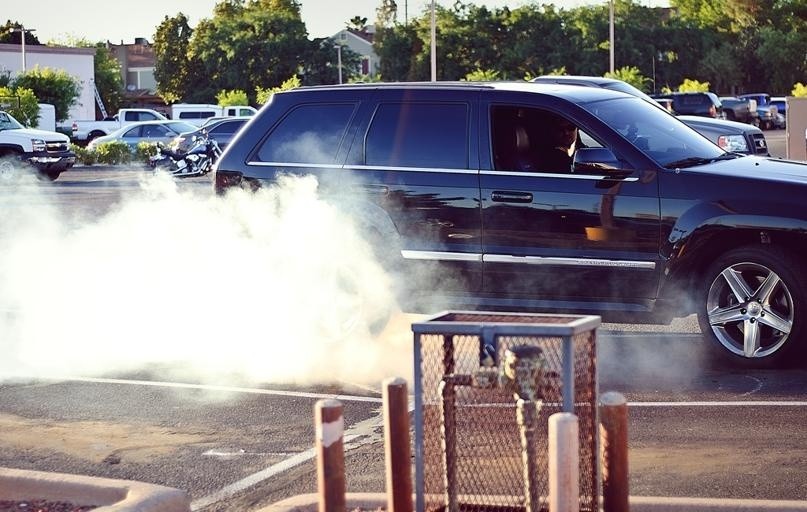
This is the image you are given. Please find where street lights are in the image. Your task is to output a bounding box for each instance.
[333,45,343,84]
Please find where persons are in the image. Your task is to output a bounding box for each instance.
[533,112,614,174]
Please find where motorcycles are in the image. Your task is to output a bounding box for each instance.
[144,127,223,181]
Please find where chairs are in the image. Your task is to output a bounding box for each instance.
[507,124,539,170]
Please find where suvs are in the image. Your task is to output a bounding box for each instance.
[0,96,76,183]
[208,80,807,372]
[531,73,771,158]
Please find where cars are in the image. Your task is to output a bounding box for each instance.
[651,92,786,129]
[69,102,258,162]
